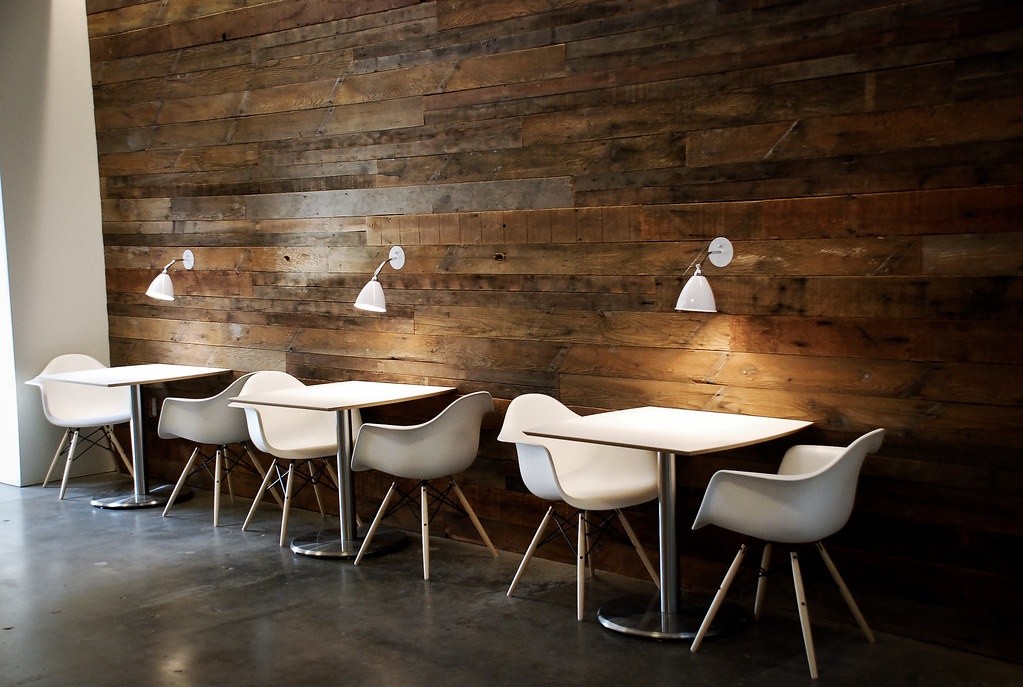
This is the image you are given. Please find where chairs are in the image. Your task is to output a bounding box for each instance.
[686,428,886,679]
[228,370,363,548]
[496,393,660,622]
[158,372,285,527]
[349,390,500,581]
[24,353,135,500]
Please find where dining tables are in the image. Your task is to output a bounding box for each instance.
[37,362,234,511]
[227,379,457,557]
[523,405,816,637]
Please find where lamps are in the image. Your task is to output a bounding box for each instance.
[673,236,734,313]
[144,249,195,301]
[353,245,406,313]
